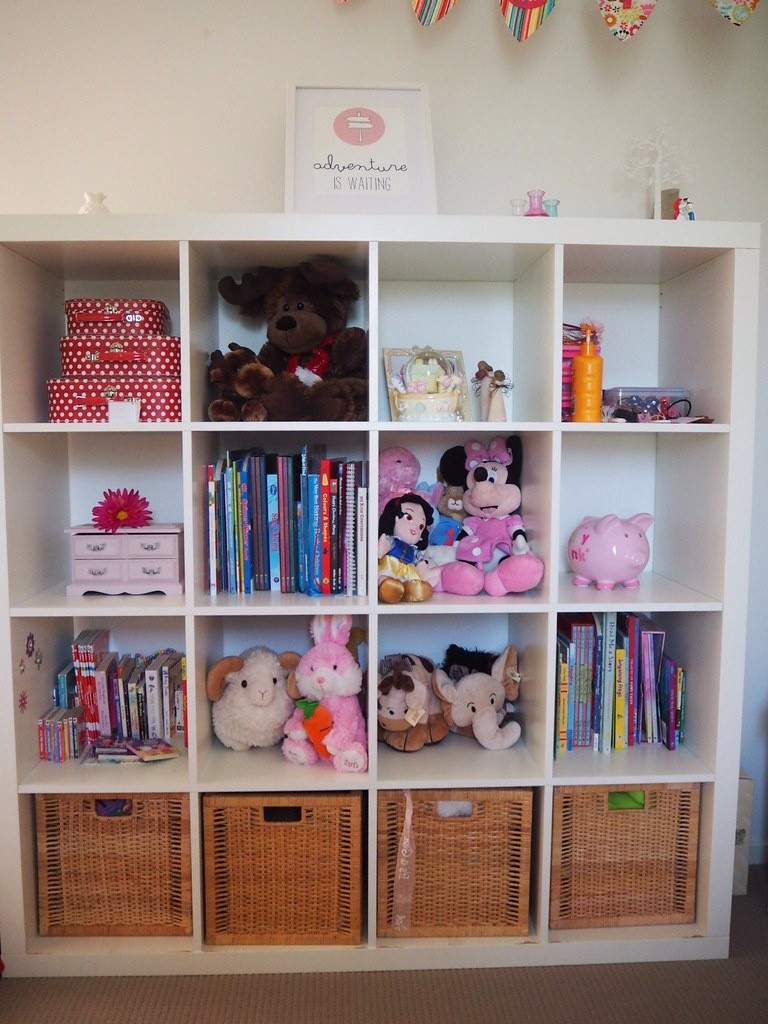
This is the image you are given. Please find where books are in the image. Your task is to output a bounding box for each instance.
[36,629,187,766]
[556,612,686,758]
[204,442,369,601]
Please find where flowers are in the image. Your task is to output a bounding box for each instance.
[90,488,154,531]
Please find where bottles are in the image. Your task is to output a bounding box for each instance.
[525,189,549,216]
[543,200,561,216]
[76,188,109,214]
[510,197,527,215]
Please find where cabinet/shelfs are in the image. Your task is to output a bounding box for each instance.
[0,217,761,983]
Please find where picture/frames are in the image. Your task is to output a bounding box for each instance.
[382,346,472,422]
[285,81,436,215]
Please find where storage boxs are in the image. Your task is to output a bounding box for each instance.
[66,524,183,596]
[731,770,754,896]
[604,386,690,417]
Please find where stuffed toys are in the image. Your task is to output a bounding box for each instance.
[204,614,367,774]
[376,643,522,753]
[206,254,370,422]
[377,435,543,604]
